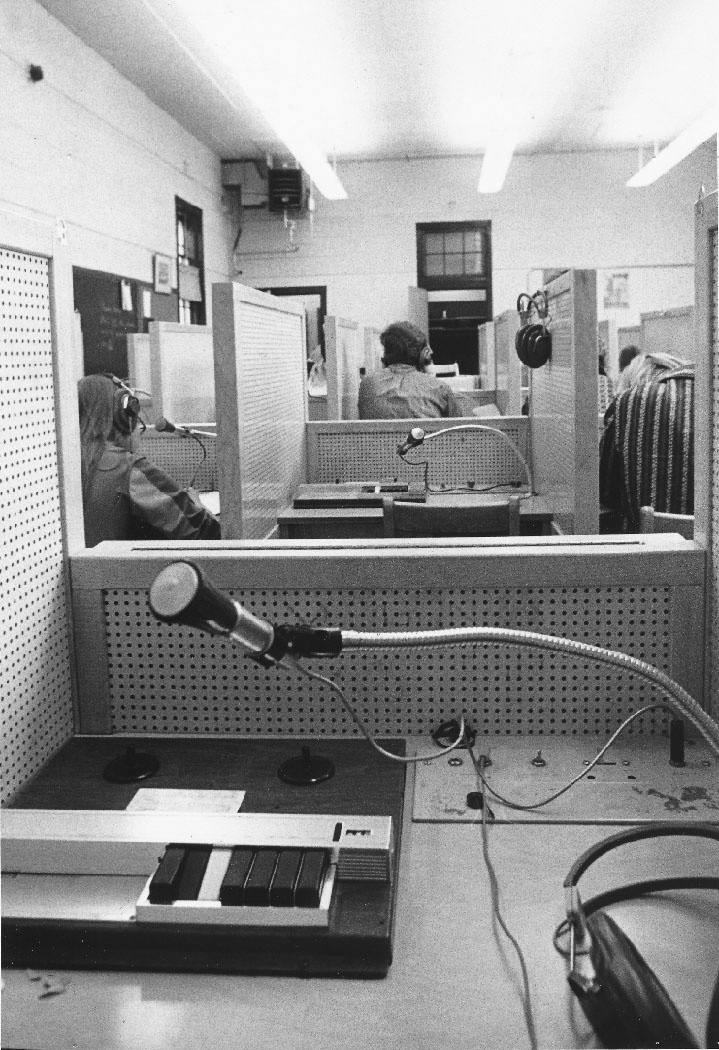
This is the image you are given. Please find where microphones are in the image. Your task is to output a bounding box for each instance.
[154,416,176,434]
[397,427,425,455]
[148,560,286,662]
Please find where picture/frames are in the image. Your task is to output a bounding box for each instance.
[153,252,172,295]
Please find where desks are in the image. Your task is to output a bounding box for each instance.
[278,484,554,538]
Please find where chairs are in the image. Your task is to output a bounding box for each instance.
[639,506,694,541]
[382,496,521,536]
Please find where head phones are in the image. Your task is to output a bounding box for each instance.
[552,820,719,1048]
[514,290,552,368]
[416,341,433,372]
[102,372,140,436]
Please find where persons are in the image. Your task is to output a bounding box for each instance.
[358,322,463,421]
[77,375,220,550]
[614,346,643,397]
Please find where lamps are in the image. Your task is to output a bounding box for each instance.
[266,103,348,201]
[477,113,517,193]
[625,102,719,189]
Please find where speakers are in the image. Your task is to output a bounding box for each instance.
[267,169,303,211]
